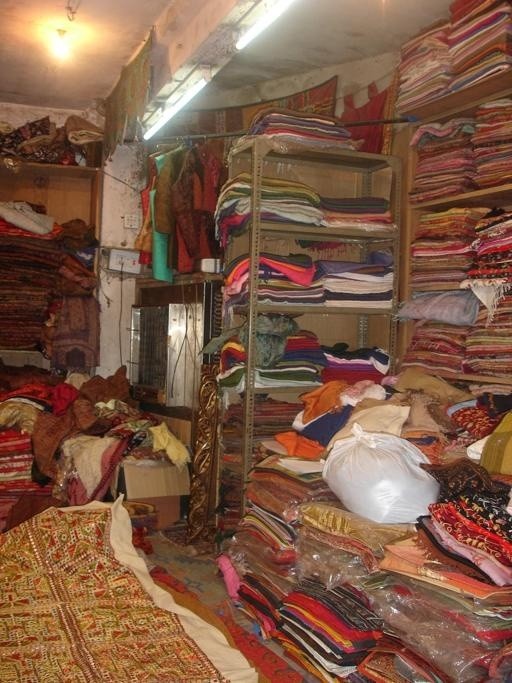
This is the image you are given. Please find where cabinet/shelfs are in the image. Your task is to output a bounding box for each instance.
[210,84,512,533]
[1,158,102,379]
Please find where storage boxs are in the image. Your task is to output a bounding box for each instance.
[111,458,192,532]
